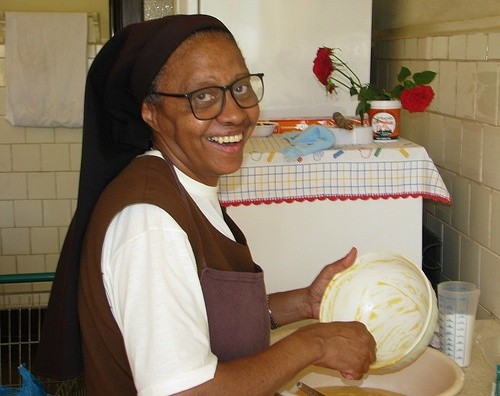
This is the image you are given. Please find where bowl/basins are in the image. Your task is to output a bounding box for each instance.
[275,346,465,396]
[250,120,279,136]
[319,250,437,376]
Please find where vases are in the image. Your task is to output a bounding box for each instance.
[364,99,402,141]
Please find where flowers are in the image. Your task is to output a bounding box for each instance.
[312,45,436,123]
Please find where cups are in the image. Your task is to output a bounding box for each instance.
[438,279,481,368]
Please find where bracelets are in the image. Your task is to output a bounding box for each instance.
[266,293,279,330]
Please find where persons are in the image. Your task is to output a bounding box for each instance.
[31,14,376,396]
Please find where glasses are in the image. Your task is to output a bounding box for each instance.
[153,73,264,121]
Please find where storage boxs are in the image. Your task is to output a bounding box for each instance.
[330,127,374,145]
